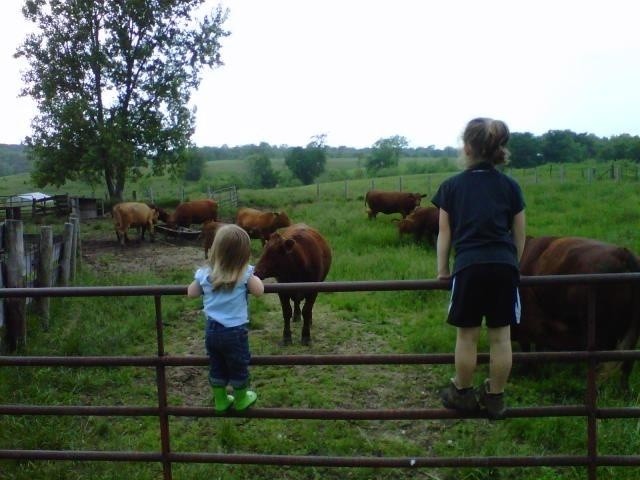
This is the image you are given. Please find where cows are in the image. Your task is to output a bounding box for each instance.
[140,203,171,241]
[202,220,251,260]
[363,190,427,222]
[510,235,640,396]
[253,222,332,347]
[237,208,291,248]
[111,202,159,249]
[168,199,219,230]
[390,205,440,248]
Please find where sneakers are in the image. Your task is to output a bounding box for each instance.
[479,378,508,421]
[438,378,480,416]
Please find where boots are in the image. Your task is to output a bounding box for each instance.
[212,384,235,414]
[232,385,258,412]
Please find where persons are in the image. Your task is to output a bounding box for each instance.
[429,118,526,416]
[184,223,265,413]
